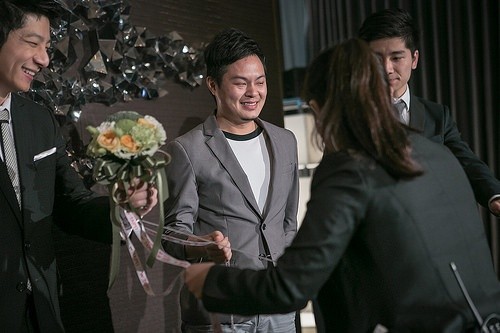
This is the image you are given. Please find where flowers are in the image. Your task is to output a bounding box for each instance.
[86,109,173,292]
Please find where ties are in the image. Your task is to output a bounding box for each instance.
[394,102,406,124]
[0,111,21,209]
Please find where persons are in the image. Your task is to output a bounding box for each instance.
[0,0,160,333]
[161,27,299,333]
[182,36,500,333]
[358,9,500,220]
